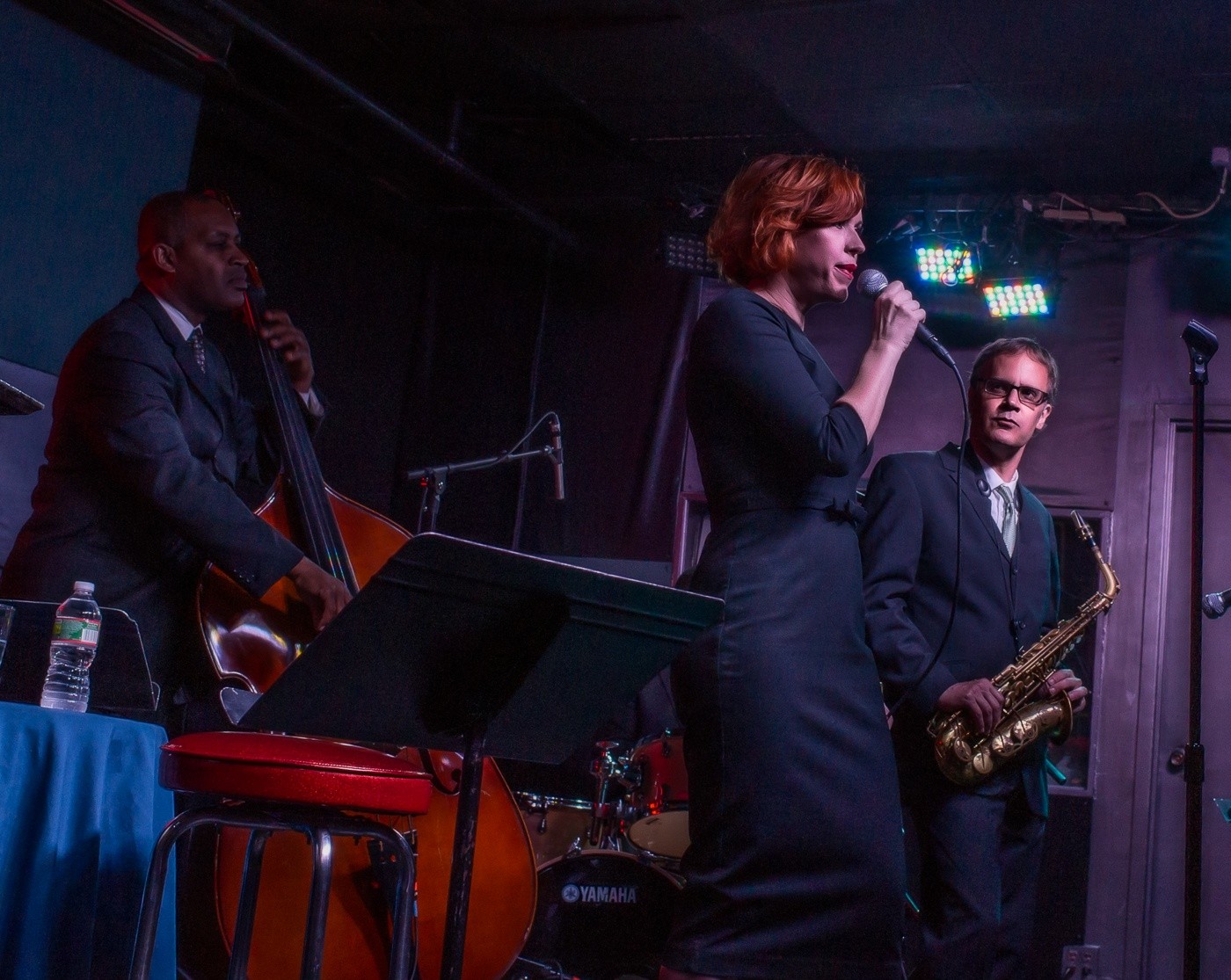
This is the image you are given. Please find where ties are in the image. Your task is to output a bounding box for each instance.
[998,485,1017,557]
[191,331,209,374]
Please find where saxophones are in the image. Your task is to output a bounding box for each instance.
[937,510,1122,785]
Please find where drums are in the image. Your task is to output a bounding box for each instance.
[622,734,691,863]
[500,848,688,980]
[508,788,615,870]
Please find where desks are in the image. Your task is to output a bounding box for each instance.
[0,700,177,980]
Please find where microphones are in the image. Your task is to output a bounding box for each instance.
[550,414,564,499]
[857,269,956,368]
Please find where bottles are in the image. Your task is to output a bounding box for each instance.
[40,580,103,712]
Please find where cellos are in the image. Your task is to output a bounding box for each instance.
[183,189,539,980]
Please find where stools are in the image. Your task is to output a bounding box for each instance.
[129,728,427,979]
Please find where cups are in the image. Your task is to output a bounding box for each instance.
[0,605,15,664]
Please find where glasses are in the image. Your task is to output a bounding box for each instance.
[979,378,1050,406]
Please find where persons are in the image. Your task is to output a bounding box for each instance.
[863,338,1088,980]
[658,156,927,980]
[0,189,354,726]
